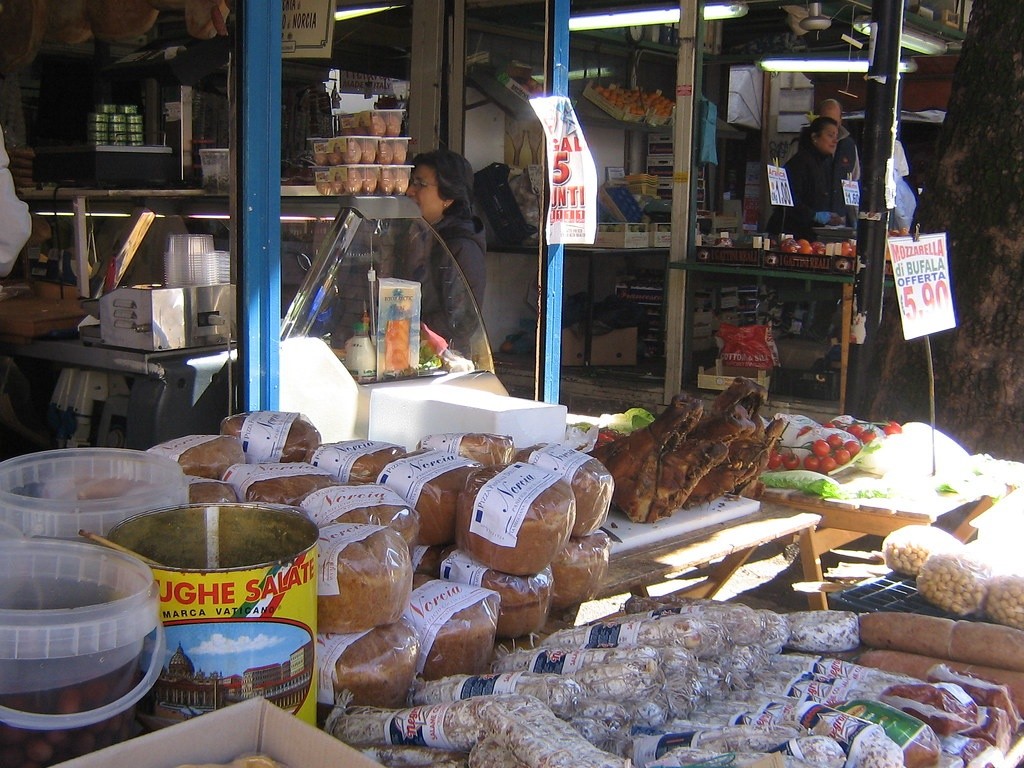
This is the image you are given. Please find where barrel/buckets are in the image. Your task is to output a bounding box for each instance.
[107,502,320,728]
[0,538,166,768]
[0,448,190,543]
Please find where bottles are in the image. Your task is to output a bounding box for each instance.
[346,321,376,385]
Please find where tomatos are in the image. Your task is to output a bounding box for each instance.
[765,419,903,474]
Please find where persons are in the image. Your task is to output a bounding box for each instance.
[765,100,861,343]
[0,123,53,277]
[396,150,486,360]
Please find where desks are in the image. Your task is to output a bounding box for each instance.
[763,469,1011,611]
[596,503,828,611]
[668,258,855,424]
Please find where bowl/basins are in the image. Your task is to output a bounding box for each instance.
[165,233,232,287]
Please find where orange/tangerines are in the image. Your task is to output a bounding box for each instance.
[595,86,674,116]
[780,237,826,255]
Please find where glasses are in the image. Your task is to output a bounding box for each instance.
[411,178,438,190]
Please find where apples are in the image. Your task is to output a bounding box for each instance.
[600,215,672,232]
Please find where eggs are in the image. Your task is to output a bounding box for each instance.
[313,113,409,196]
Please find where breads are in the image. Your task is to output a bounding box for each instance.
[73,411,616,711]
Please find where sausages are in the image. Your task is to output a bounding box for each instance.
[328,602,1024,768]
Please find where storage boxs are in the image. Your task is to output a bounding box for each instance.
[743,162,761,232]
[646,133,705,211]
[561,321,638,368]
[695,243,762,267]
[369,384,568,447]
[649,223,703,247]
[763,248,856,275]
[49,696,385,767]
[691,282,821,392]
[496,67,542,103]
[583,79,672,124]
[305,108,416,197]
[593,222,651,249]
[933,9,961,29]
[630,183,657,198]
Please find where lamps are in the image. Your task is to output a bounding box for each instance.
[568,0,749,32]
[852,15,948,56]
[756,54,918,73]
